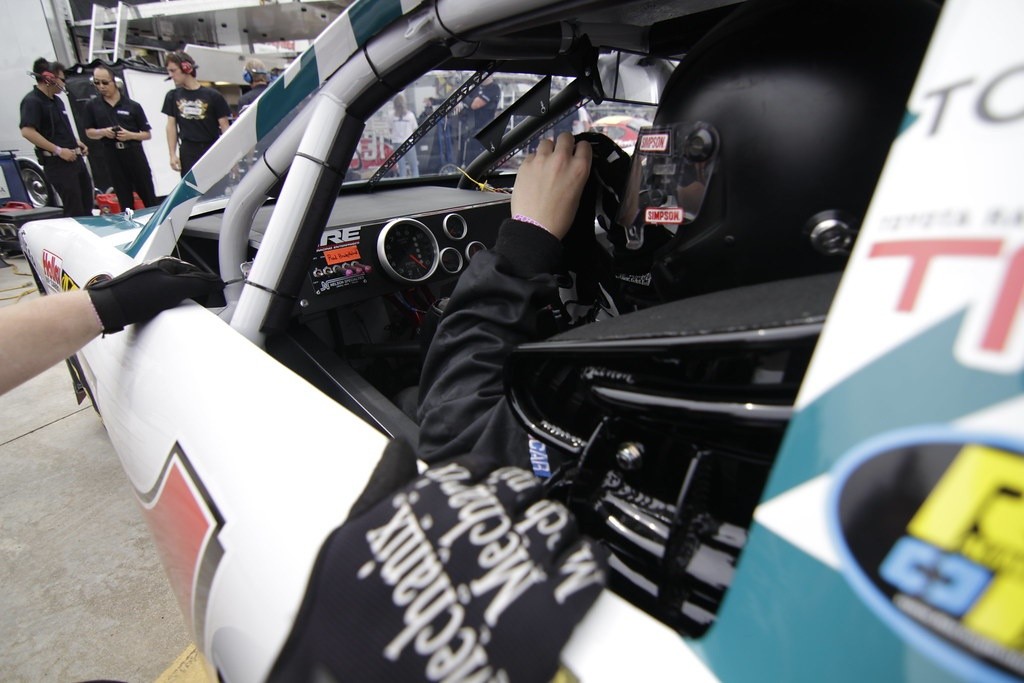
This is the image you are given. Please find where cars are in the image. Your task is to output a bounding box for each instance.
[18,0,1024,683]
[588,116,653,157]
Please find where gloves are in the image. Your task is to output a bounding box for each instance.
[87,257,227,334]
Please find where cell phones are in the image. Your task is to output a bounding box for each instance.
[112,126,121,131]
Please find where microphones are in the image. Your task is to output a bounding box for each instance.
[164,76,172,81]
[52,80,69,95]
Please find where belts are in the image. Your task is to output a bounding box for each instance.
[43,148,83,158]
[105,140,141,148]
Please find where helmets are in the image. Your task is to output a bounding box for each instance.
[651,4,919,290]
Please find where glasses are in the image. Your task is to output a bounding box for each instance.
[56,75,65,83]
[92,76,114,85]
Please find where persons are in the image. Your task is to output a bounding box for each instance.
[0,255,226,396]
[19,58,94,217]
[236,58,271,116]
[415,0,941,487]
[160,51,231,181]
[84,64,158,213]
[554,101,590,142]
[461,72,501,129]
[391,93,419,177]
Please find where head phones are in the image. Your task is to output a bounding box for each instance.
[42,62,56,85]
[173,52,194,73]
[89,66,124,89]
[242,68,273,83]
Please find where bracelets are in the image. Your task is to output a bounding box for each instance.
[55,146,61,155]
[96,129,102,139]
